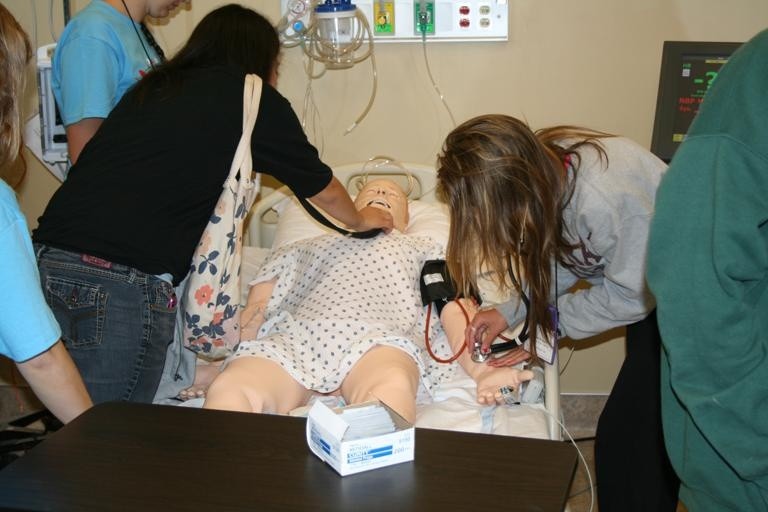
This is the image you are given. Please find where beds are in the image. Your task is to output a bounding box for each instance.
[155,163,571,512]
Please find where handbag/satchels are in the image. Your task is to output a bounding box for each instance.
[180,176,255,362]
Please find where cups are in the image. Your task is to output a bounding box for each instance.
[313,1,356,70]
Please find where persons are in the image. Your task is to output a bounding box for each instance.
[174,176,536,427]
[29,4,394,404]
[47,0,194,170]
[639,31,767,512]
[434,109,679,510]
[0,2,95,428]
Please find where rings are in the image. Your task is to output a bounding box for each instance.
[470,327,477,332]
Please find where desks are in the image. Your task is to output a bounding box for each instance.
[0,402,580,512]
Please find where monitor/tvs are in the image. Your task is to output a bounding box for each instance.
[650,41,746,164]
